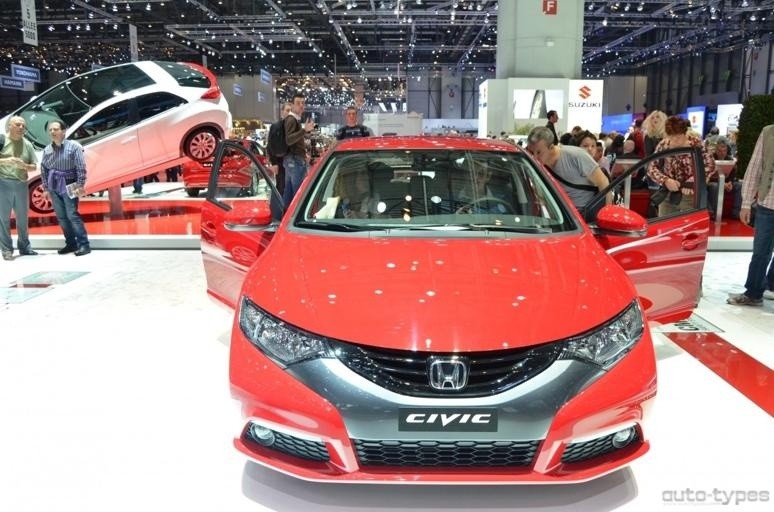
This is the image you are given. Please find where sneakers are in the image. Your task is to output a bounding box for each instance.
[763,289,774,300]
[18,249,39,256]
[727,295,764,307]
[2,250,15,260]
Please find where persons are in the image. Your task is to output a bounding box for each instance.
[86,165,181,197]
[455,158,508,214]
[527,110,774,308]
[270,102,293,223]
[313,192,381,219]
[0,116,38,260]
[40,119,91,256]
[282,93,316,214]
[336,106,369,140]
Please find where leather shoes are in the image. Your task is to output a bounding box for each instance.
[74,243,91,256]
[58,243,78,254]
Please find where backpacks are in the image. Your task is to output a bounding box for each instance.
[268,114,298,158]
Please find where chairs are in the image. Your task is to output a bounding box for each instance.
[410,177,432,211]
[345,162,409,221]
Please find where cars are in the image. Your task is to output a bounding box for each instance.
[181,135,269,196]
[197,132,717,490]
[0,55,236,216]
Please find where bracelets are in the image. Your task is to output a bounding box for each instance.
[367,211,371,219]
